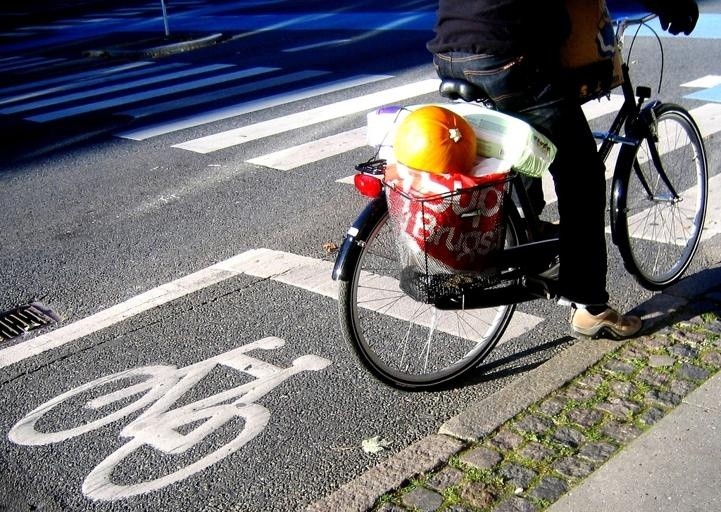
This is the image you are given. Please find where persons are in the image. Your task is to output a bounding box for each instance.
[426,0,698,339]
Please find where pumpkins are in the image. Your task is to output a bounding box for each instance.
[393,104,478,174]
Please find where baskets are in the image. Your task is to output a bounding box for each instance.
[381,171,515,302]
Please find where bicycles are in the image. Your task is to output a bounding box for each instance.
[331,10,708,391]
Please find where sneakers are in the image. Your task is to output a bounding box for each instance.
[568,307,642,342]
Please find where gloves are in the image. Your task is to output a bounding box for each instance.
[649,2,699,35]
